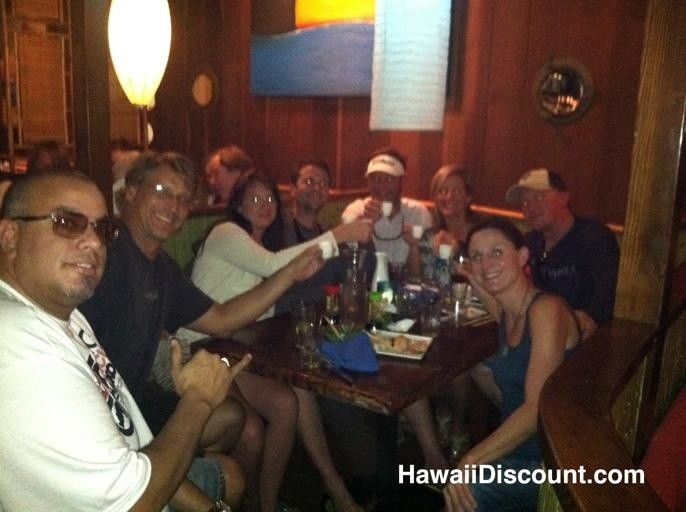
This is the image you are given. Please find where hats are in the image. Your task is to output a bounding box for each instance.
[504,167,566,204]
[363,153,404,178]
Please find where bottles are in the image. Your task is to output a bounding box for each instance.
[292,249,366,367]
[369,250,394,317]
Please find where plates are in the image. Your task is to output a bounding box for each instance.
[364,329,434,362]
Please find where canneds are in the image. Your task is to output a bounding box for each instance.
[321,284,341,327]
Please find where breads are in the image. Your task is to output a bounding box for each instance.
[407,339,428,355]
[391,334,408,353]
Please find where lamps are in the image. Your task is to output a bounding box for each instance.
[369,0,452,130]
[108,0,172,151]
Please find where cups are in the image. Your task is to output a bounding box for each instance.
[381,202,393,215]
[318,240,333,260]
[403,274,476,338]
[411,225,424,240]
[438,244,453,261]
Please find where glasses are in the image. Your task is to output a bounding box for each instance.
[242,194,275,206]
[142,181,195,211]
[9,210,120,246]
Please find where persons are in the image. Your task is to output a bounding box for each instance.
[1,136,622,511]
